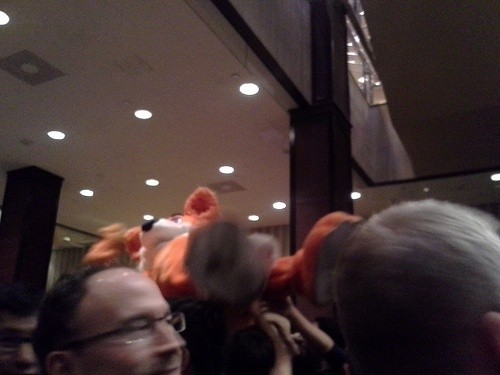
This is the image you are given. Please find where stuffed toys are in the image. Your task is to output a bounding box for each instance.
[80,186,365,309]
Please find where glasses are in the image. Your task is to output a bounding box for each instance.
[72,312,186,347]
[1,333,34,351]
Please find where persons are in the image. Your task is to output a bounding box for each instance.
[0,199,500,375]
